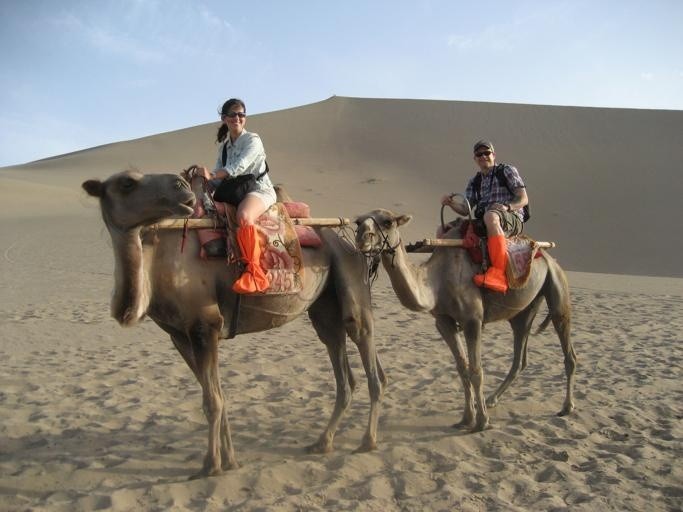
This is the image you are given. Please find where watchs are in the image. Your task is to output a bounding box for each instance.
[210,172,217,180]
[503,203,511,212]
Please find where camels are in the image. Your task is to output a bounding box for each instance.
[81,170,387,482]
[349,208,577,432]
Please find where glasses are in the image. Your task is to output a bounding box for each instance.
[225,111,246,118]
[474,151,493,157]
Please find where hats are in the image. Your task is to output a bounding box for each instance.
[474,139,494,151]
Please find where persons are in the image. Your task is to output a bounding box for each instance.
[196,98,276,293]
[436,138,530,293]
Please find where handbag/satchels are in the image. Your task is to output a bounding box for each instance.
[212,173,261,204]
